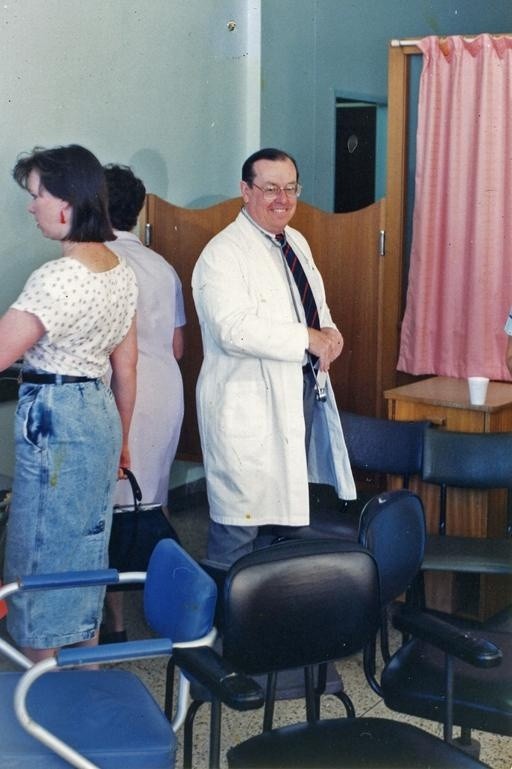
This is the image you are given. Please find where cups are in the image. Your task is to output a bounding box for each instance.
[468,375,491,406]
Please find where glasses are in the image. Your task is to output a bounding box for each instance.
[250,181,302,200]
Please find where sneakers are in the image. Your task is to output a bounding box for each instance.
[97,624,130,646]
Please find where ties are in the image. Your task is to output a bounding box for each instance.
[276,233,324,332]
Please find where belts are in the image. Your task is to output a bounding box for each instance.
[13,371,96,383]
[299,357,320,374]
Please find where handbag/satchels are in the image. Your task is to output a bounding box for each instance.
[105,468,182,593]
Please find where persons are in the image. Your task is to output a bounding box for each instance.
[102,163,186,643]
[190,148,359,565]
[1,144,138,674]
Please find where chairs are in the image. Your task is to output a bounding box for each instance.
[165,536,355,769]
[356,489,512,759]
[411,422,511,616]
[280,410,433,647]
[0,538,217,769]
[171,540,505,768]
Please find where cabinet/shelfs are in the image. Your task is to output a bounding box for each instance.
[383,376,512,624]
